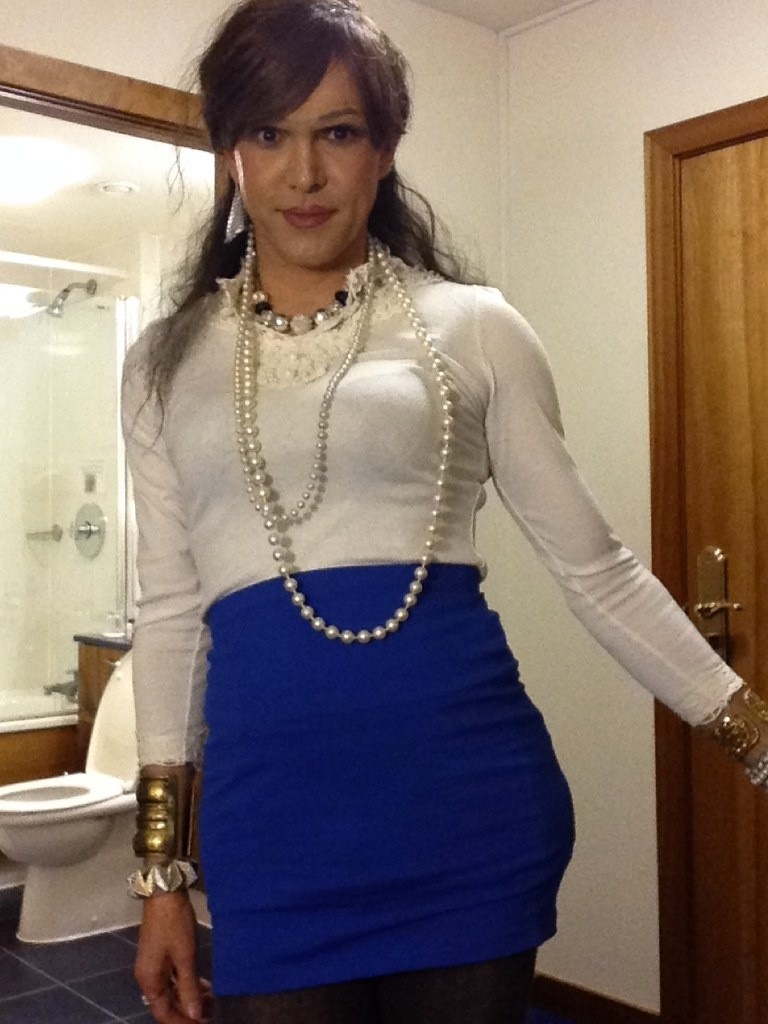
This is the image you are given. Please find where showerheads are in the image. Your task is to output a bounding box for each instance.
[46,288,71,320]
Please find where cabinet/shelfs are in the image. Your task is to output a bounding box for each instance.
[73,642,202,893]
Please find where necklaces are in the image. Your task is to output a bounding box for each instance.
[232,218,453,643]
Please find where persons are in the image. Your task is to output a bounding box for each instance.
[120,0,768,1024]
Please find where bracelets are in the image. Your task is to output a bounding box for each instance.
[711,688,768,785]
[125,777,199,899]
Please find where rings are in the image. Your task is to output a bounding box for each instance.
[142,987,166,1005]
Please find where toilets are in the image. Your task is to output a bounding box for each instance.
[0,646,145,945]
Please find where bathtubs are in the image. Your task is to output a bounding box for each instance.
[0,687,80,786]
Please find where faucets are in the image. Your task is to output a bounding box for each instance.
[43,668,79,704]
